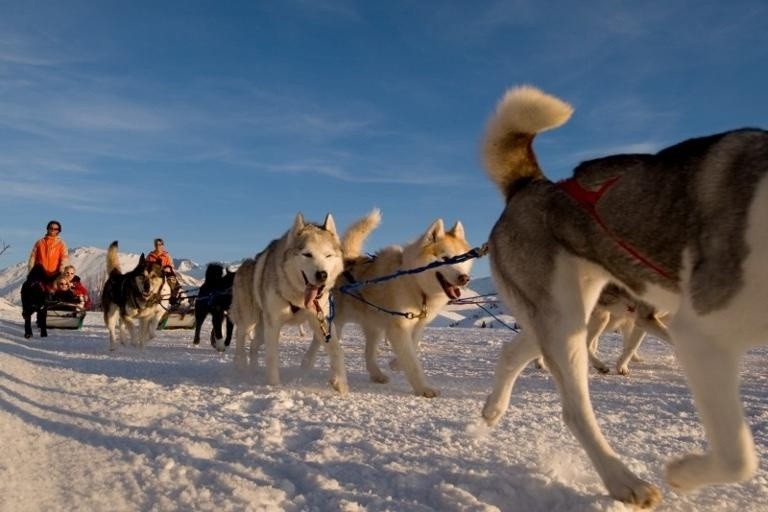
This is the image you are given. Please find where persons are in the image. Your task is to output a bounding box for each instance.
[145,238,174,272]
[28,220,92,310]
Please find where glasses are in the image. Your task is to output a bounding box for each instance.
[50,228,59,230]
[65,272,74,276]
[58,283,69,285]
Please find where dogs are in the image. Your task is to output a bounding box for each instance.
[193,263,250,348]
[469,89,768,510]
[299,206,475,391]
[99,239,172,352]
[230,213,353,398]
